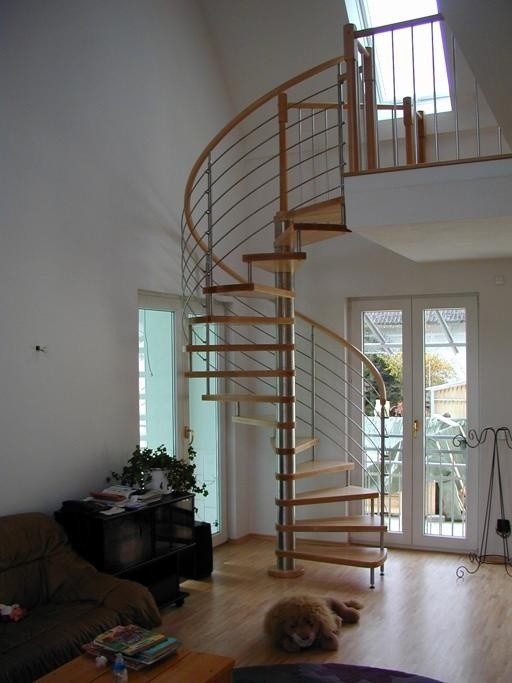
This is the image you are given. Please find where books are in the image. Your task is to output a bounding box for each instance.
[80,484,164,516]
[81,623,184,672]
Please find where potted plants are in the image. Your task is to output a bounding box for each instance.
[106,444,209,497]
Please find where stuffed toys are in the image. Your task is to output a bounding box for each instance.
[262,592,365,652]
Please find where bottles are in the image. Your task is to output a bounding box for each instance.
[112,653,128,683]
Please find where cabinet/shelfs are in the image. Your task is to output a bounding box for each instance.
[63,490,195,609]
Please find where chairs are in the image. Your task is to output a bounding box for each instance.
[1,512,148,682]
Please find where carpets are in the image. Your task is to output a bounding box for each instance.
[233,663,445,683]
